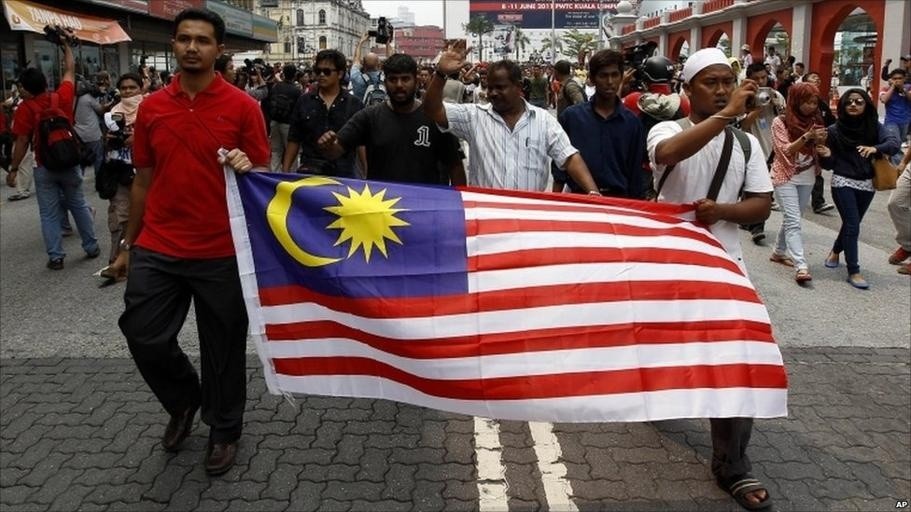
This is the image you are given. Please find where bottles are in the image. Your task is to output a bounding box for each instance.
[899,53,911,61]
[740,43,751,52]
[682,47,733,84]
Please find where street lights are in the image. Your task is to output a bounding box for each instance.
[708,127,732,205]
[873,158,897,190]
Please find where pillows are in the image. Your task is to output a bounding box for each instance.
[642,56,675,83]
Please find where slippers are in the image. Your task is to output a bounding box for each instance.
[25,91,86,173]
[362,71,390,107]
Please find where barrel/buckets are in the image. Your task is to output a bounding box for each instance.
[795,268,813,282]
[716,470,773,511]
[769,253,795,267]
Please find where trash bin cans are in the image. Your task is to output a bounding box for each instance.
[8,164,20,172]
[119,239,130,250]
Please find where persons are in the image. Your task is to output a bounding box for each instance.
[267,65,305,171]
[803,72,834,212]
[887,128,911,281]
[738,44,751,71]
[620,53,692,132]
[509,56,591,102]
[216,54,237,84]
[794,62,803,90]
[103,9,271,475]
[295,60,317,90]
[522,65,548,108]
[93,74,151,278]
[784,55,805,86]
[320,55,465,186]
[67,74,105,192]
[136,65,175,93]
[470,70,489,103]
[234,75,266,100]
[419,67,427,85]
[553,58,586,193]
[830,58,879,88]
[8,83,40,200]
[815,89,896,287]
[349,31,392,179]
[766,45,789,79]
[426,38,603,197]
[647,50,779,509]
[7,41,99,268]
[281,49,368,177]
[247,67,263,103]
[460,58,485,89]
[881,55,909,79]
[767,82,827,284]
[883,68,908,160]
[549,50,652,200]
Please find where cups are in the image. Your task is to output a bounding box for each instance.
[313,67,339,77]
[845,97,866,106]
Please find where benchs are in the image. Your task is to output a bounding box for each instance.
[216,147,790,423]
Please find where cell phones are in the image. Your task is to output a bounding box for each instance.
[751,88,772,107]
[242,59,273,80]
[77,80,108,98]
[369,17,393,44]
[624,41,657,90]
[41,25,82,47]
[108,112,130,150]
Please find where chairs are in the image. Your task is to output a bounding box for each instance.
[61,227,74,238]
[738,224,750,230]
[896,260,911,275]
[768,201,780,211]
[750,232,766,242]
[7,188,31,201]
[90,207,98,222]
[814,202,834,213]
[888,249,910,265]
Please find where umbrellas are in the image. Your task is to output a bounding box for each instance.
[159,394,196,454]
[825,248,839,267]
[100,264,124,280]
[86,244,101,259]
[847,273,869,289]
[200,428,239,478]
[46,257,64,271]
